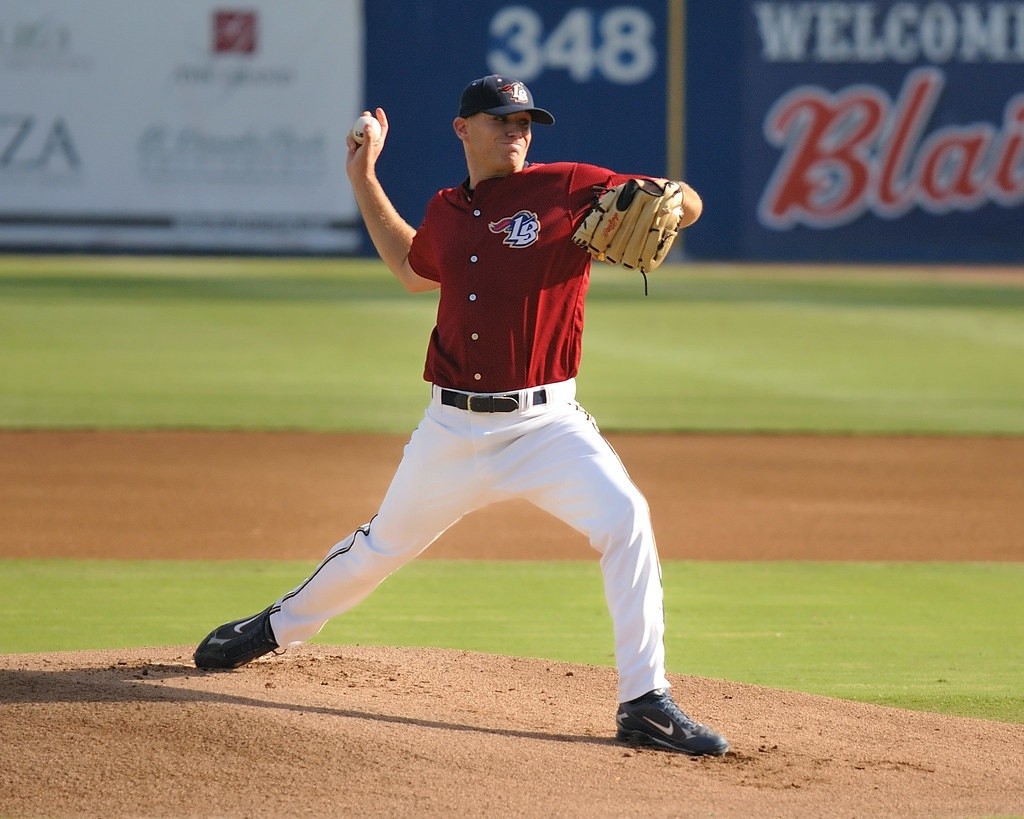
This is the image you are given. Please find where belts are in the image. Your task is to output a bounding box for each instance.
[442,389,547,413]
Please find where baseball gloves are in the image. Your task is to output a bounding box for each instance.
[569,176,686,296]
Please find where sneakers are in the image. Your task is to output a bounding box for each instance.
[194,602,285,668]
[615,688,730,754]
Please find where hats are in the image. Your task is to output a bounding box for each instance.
[459,74,555,124]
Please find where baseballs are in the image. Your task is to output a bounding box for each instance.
[352,115,382,144]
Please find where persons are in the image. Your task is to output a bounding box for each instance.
[194,74,728,756]
